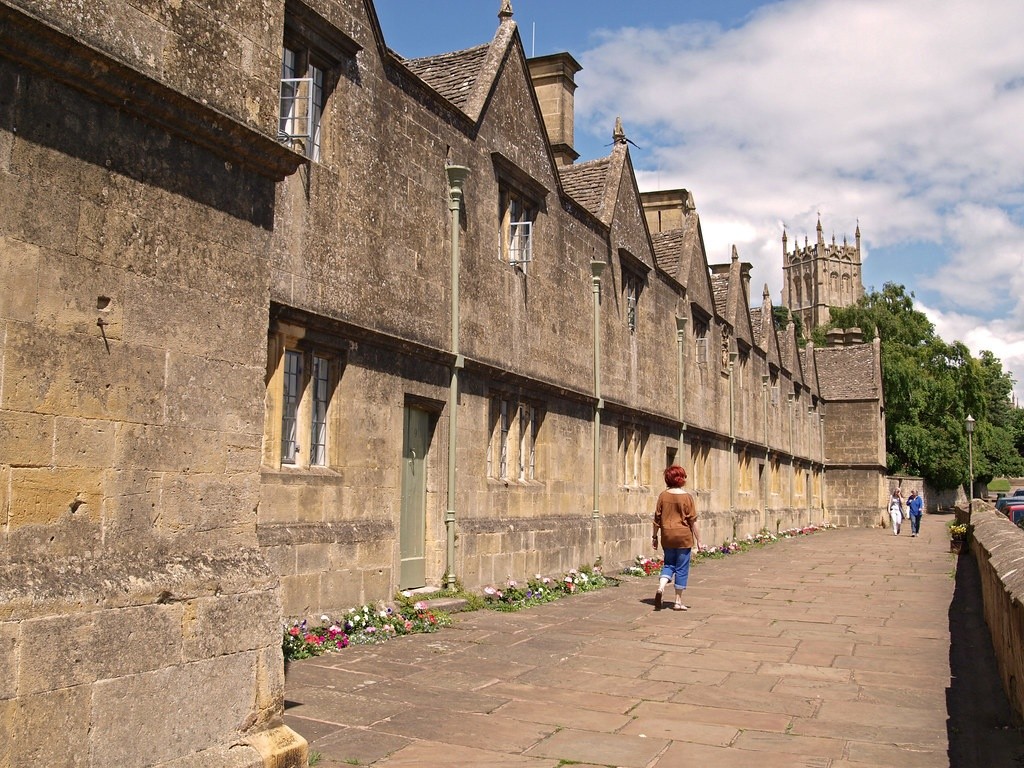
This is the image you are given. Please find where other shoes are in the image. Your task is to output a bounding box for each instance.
[894,533,897,536]
[897,530,900,534]
[654,587,663,611]
[916,534,919,537]
[673,602,688,611]
[912,533,915,537]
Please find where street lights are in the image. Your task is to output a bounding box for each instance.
[964,414,975,500]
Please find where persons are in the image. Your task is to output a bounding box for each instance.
[888,487,904,536]
[652,465,701,611]
[906,489,923,537]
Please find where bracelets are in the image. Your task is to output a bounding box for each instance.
[652,536,657,539]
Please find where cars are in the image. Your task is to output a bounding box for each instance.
[996,487,1024,526]
